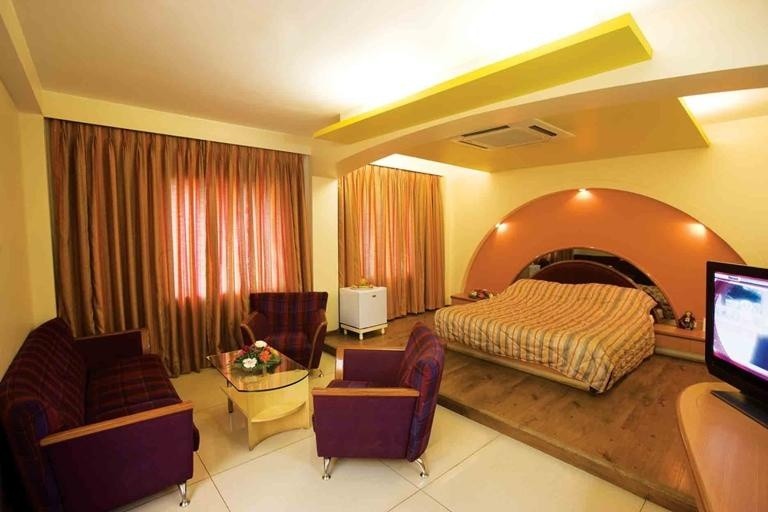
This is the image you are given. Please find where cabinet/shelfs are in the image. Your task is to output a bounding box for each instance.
[339,285,388,342]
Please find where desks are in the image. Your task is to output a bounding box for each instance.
[675,379,767,512]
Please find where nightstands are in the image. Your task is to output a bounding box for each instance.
[654,321,707,362]
[449,292,482,308]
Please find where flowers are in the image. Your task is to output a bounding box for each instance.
[229,340,281,376]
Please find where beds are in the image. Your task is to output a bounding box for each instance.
[434,256,657,397]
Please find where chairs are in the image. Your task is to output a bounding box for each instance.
[312,321,443,480]
[236,289,328,379]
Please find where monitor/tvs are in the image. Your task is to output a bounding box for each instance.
[704,260,768,430]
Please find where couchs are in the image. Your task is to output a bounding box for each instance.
[1,316,199,511]
